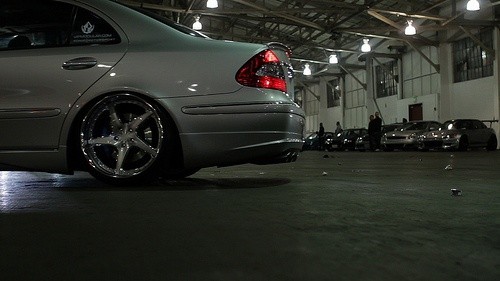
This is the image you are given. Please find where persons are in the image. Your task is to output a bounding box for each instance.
[317,123,324,151]
[367,112,382,151]
[336,121,341,132]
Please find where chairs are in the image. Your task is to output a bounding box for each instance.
[8,35,31,50]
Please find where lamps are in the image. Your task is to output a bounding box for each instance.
[328,52,338,64]
[361,37,371,52]
[192,13,202,30]
[303,62,312,75]
[405,18,416,35]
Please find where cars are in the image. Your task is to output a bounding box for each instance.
[417,119,498,151]
[304,122,416,152]
[381,121,443,152]
[0,0,306,185]
